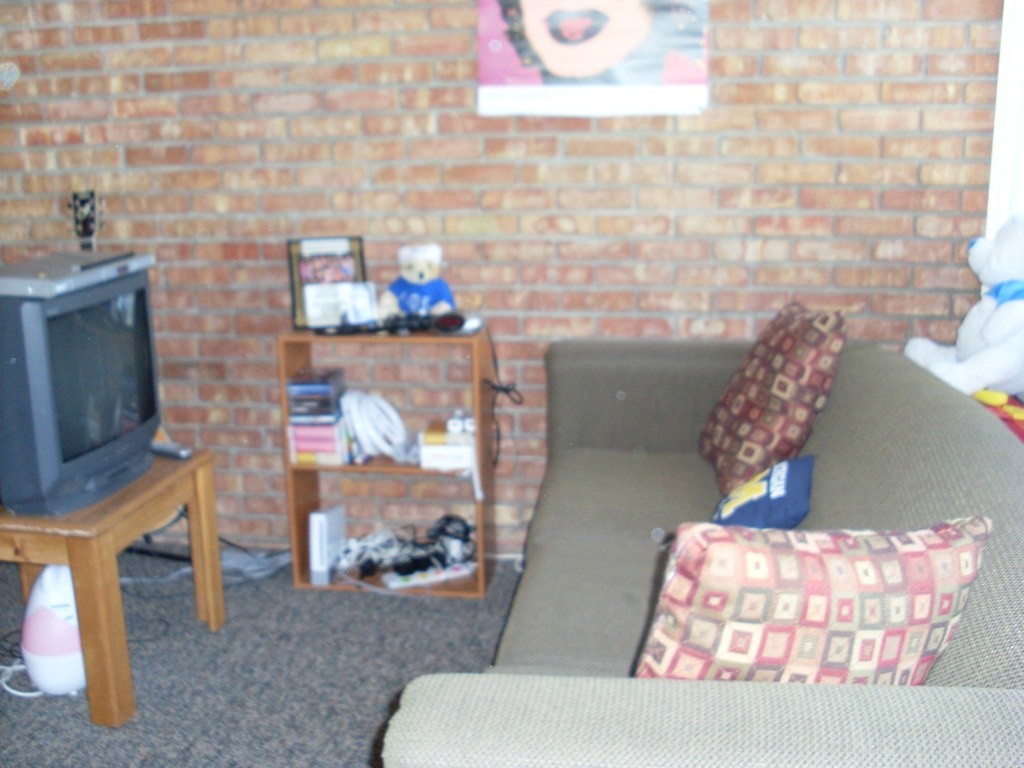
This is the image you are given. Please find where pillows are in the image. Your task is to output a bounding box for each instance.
[699,300,848,496]
[633,514,992,688]
[708,456,816,527]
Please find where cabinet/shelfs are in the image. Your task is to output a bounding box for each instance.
[279,328,491,596]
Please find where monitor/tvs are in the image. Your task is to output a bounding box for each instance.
[0,270,162,518]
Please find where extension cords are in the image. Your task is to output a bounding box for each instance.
[382,562,476,590]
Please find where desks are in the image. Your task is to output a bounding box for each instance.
[0,449,225,726]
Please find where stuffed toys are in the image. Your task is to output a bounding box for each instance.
[378,244,465,333]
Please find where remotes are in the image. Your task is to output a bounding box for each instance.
[151,443,194,460]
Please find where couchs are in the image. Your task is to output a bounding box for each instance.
[379,339,1024,768]
